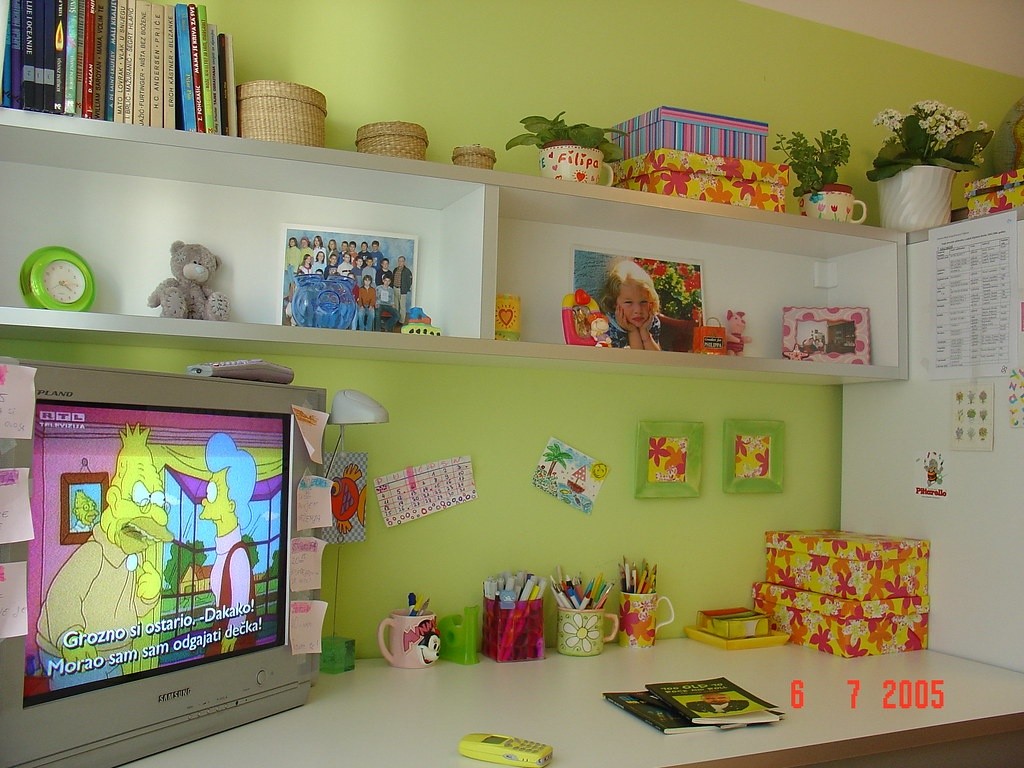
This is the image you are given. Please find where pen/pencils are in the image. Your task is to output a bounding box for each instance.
[548,565,582,609]
[419,599,430,616]
[581,573,614,610]
[617,555,657,594]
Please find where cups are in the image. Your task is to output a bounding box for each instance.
[556,606,619,656]
[616,592,675,647]
[481,598,545,662]
[378,608,442,668]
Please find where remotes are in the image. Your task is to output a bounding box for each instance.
[188,359,295,385]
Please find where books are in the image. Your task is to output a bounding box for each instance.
[0,0,240,137]
[600,675,786,737]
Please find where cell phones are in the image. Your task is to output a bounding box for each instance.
[457,733,553,768]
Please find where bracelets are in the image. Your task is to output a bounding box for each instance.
[643,334,652,342]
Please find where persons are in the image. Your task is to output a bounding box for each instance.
[802,337,827,354]
[685,692,749,713]
[597,261,663,351]
[284,235,412,332]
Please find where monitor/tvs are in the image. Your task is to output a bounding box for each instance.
[0,356,319,768]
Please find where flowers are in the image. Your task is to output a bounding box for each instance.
[865,100,996,182]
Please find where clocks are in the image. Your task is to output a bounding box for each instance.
[19,245,95,311]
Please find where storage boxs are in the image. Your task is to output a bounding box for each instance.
[610,106,769,162]
[766,530,929,600]
[610,148,789,215]
[752,581,929,658]
[964,167,1024,216]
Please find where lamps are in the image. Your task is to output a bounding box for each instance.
[324,388,391,478]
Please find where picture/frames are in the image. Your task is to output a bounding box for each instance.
[721,418,785,495]
[780,305,870,364]
[634,421,702,498]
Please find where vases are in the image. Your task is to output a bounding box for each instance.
[876,164,956,231]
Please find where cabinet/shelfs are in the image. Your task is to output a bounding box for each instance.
[0,104,910,384]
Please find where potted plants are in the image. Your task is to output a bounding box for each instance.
[507,111,629,184]
[771,130,869,225]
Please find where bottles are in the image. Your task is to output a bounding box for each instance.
[292,274,356,331]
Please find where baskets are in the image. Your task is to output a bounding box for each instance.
[354,121,429,160]
[236,79,329,148]
[451,144,496,170]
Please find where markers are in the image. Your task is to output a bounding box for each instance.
[410,596,423,616]
[484,570,547,662]
[408,593,416,613]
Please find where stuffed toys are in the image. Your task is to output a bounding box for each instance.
[145,239,232,320]
[587,313,612,347]
[726,310,752,356]
